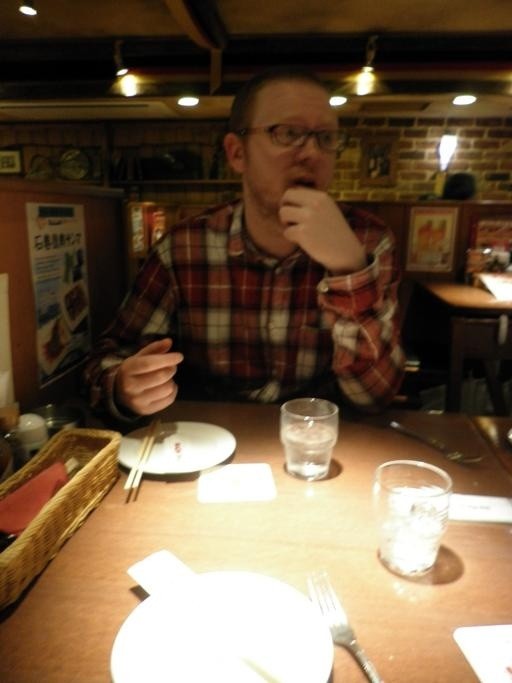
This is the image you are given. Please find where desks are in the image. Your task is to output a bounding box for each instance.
[419,278,512,415]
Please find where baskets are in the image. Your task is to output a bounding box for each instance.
[0,424,123,611]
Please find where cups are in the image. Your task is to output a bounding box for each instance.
[280,398,340,482]
[373,460,452,577]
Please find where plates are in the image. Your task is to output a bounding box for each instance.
[116,422,237,478]
[110,573,334,678]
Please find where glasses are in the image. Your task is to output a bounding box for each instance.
[234,122,346,152]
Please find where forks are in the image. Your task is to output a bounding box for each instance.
[299,565,381,677]
[390,418,492,466]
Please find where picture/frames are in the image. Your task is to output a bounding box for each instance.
[405,206,461,273]
[1,146,24,176]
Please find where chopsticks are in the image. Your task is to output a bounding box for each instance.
[122,417,155,493]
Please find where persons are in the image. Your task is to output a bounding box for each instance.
[84,67,407,434]
[442,173,475,200]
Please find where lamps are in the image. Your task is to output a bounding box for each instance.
[19,0,38,17]
[112,38,129,77]
